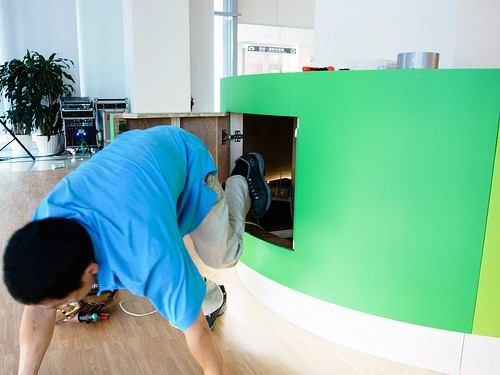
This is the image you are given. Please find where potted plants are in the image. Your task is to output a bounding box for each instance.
[0,49,75,156]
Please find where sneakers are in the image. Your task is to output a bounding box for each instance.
[205,285,227,331]
[235,152,271,213]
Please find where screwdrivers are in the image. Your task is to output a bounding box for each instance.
[60,312,111,324]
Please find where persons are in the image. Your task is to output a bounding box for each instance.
[2,125,271,375]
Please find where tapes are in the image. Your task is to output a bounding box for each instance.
[396,51,438,68]
